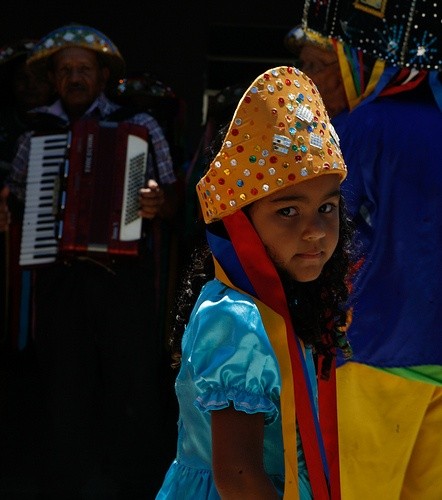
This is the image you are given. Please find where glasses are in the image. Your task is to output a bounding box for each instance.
[292,57,338,73]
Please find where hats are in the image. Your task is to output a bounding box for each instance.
[0,38,39,63]
[108,72,177,98]
[24,23,129,79]
[210,81,252,113]
[197,67,348,225]
[301,0,442,72]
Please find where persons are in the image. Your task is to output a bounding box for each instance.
[156,66,347,500]
[290,26,441,499]
[8,21,176,423]
